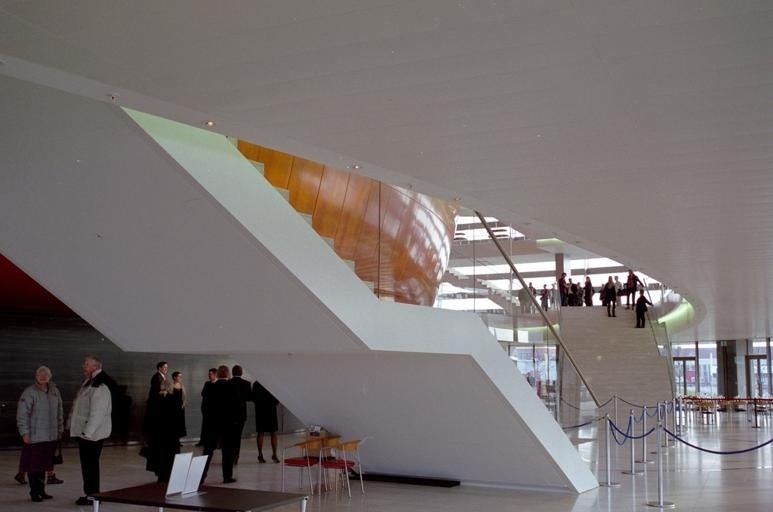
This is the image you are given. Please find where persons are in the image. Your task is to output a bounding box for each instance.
[517,269,622,311]
[139,362,252,485]
[252,380,281,464]
[16,365,64,502]
[14,442,63,486]
[635,290,653,328]
[66,355,111,505]
[623,268,643,311]
[602,276,616,317]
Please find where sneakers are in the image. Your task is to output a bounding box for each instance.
[76,496,99,505]
[223,478,235,483]
[47,475,64,484]
[14,474,27,484]
[30,491,52,502]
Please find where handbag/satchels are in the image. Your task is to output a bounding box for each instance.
[54,455,63,464]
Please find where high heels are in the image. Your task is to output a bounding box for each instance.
[272,456,280,463]
[258,455,266,463]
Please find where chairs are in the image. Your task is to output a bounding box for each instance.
[281,428,364,498]
[681,393,772,424]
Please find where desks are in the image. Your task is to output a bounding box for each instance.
[84,479,309,512]
